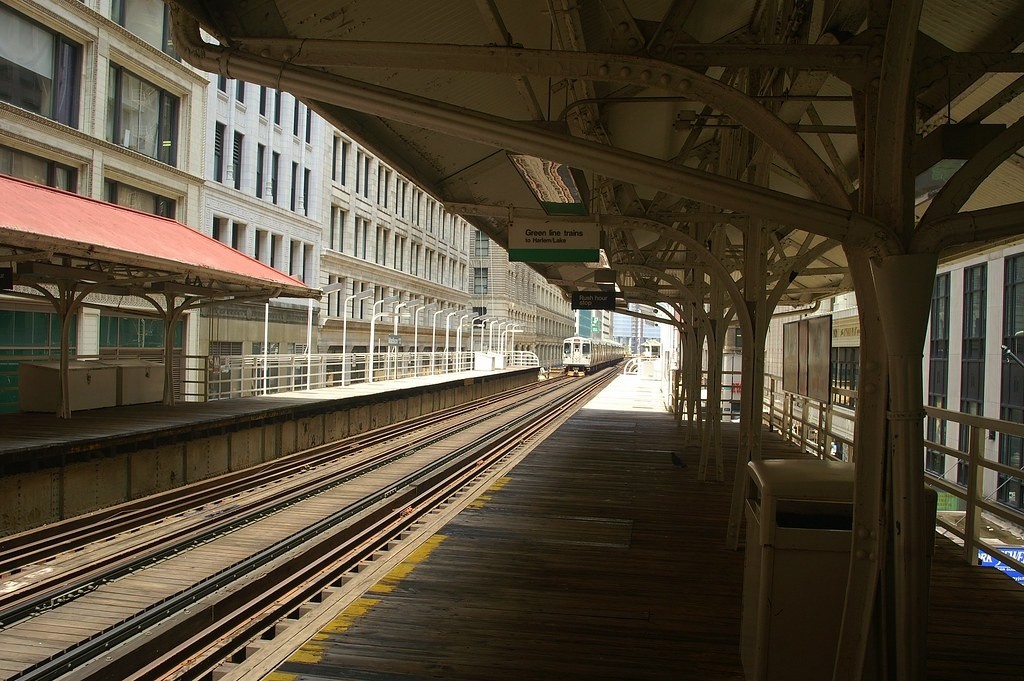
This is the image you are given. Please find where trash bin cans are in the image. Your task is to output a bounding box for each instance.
[740,458,938,681]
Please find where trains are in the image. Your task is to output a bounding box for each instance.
[562,336,626,377]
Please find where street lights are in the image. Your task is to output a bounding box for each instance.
[369,295,400,384]
[263,274,302,397]
[432,307,455,374]
[413,302,440,377]
[470,314,526,370]
[340,288,374,387]
[458,311,480,369]
[394,298,421,381]
[305,283,343,391]
[445,307,469,374]
